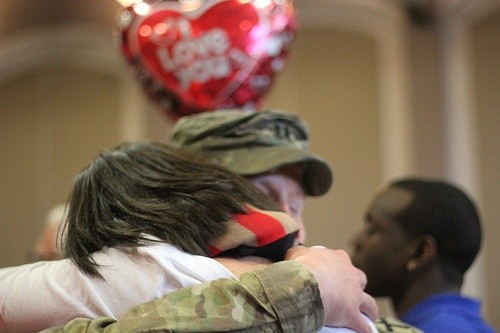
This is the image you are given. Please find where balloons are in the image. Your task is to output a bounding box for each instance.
[114,0,299,122]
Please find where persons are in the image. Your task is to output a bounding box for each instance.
[32,109,423,333]
[345,175,493,332]
[0,136,381,332]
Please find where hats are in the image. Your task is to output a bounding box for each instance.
[165,107,333,197]
